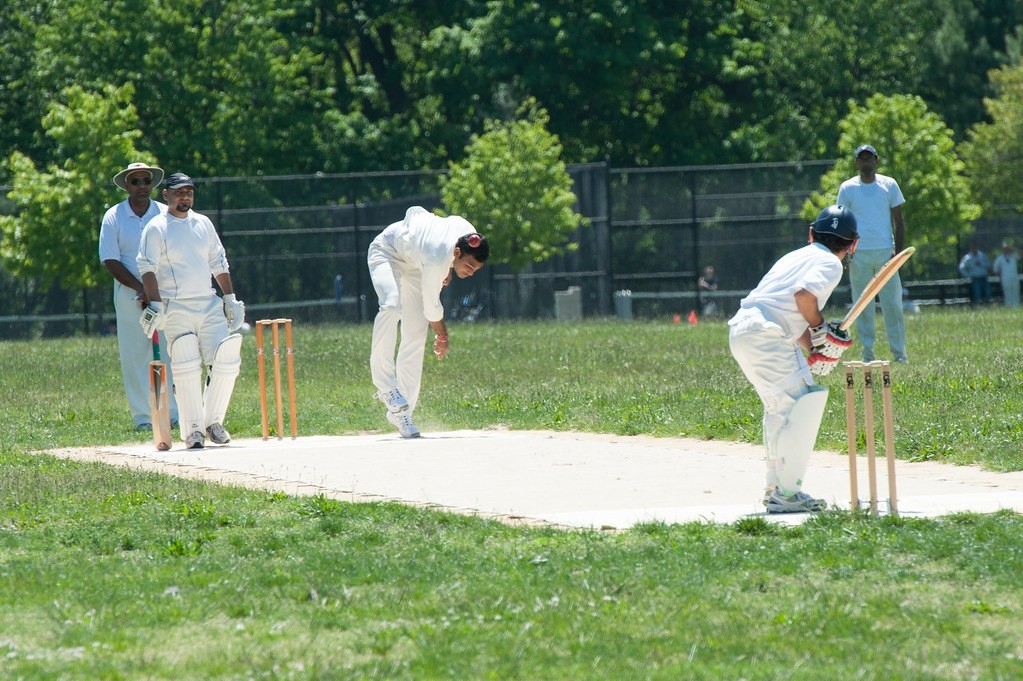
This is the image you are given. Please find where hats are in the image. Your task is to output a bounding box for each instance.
[856,145,876,157]
[113,162,164,191]
[165,173,196,190]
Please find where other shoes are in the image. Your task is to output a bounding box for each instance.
[137,423,152,430]
[171,422,181,430]
[862,355,878,363]
[895,356,908,364]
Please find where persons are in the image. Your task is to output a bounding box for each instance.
[836,144,908,362]
[367,206,489,438]
[724,205,859,516]
[958,240,991,307]
[697,265,718,317]
[99,163,179,429]
[993,241,1021,306]
[135,172,244,448]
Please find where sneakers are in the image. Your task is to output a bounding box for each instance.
[385,411,420,438]
[763,486,827,513]
[377,389,409,413]
[206,423,231,444]
[186,431,204,449]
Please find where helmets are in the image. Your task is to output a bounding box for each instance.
[811,205,860,240]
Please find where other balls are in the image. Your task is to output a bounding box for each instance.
[468,234,482,248]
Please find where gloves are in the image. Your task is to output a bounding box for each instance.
[222,294,245,332]
[140,301,165,338]
[808,318,830,345]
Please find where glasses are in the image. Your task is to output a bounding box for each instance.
[128,177,152,185]
